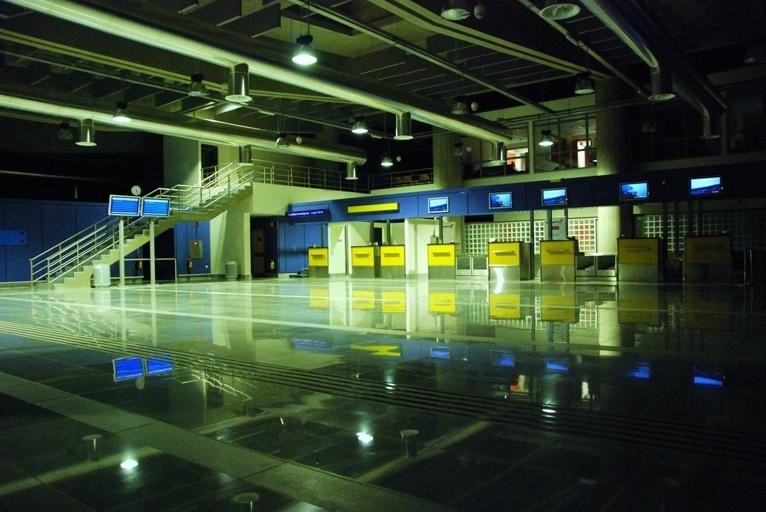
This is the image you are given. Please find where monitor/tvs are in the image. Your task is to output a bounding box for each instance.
[108,195,140,216]
[141,197,170,217]
[688,176,722,196]
[489,192,513,209]
[619,181,649,202]
[541,187,567,205]
[428,197,449,213]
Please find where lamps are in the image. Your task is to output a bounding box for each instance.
[73,97,131,151]
[185,2,319,104]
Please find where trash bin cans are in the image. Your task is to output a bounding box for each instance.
[225,261,238,281]
[93,264,111,288]
